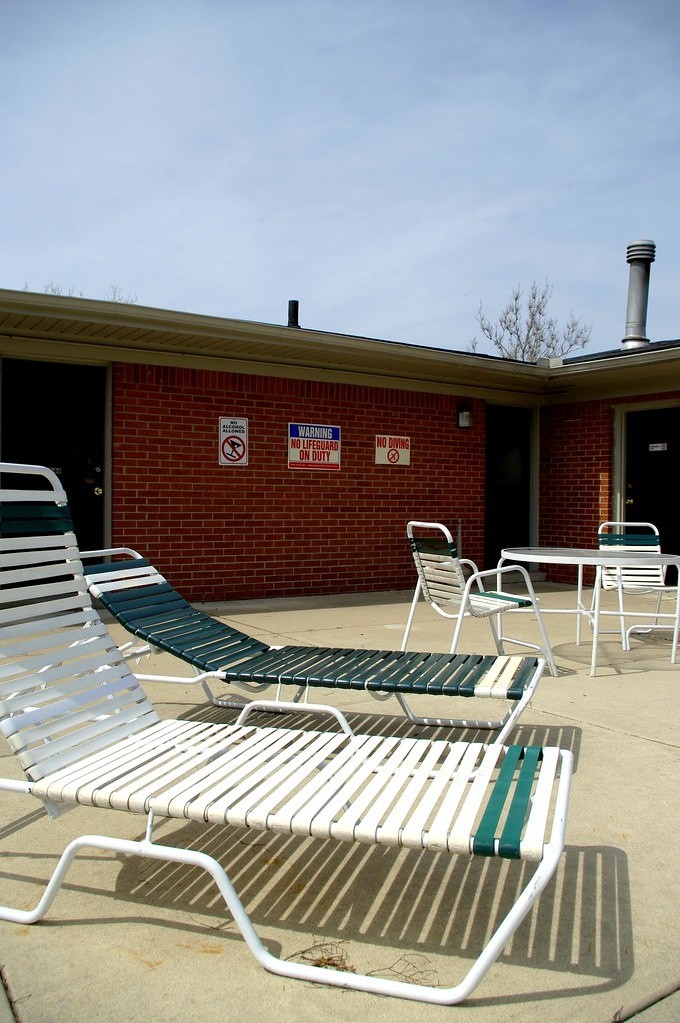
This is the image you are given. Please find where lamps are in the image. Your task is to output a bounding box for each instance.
[457,400,473,429]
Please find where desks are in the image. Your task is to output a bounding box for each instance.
[497,549,680,678]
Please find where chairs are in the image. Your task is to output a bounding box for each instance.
[0,459,574,1003]
[585,522,680,665]
